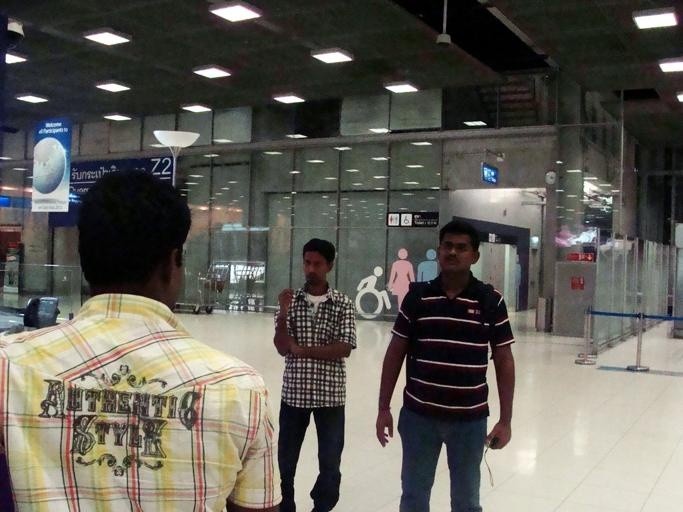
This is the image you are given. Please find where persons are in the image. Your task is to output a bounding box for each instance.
[0,170,284,512]
[376,219,515,512]
[274,239,357,512]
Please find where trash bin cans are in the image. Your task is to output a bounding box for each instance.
[535,297,552,332]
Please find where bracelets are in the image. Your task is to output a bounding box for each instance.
[276,314,288,326]
[379,407,390,411]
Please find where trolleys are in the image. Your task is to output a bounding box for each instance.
[173,261,230,314]
[226,263,261,312]
